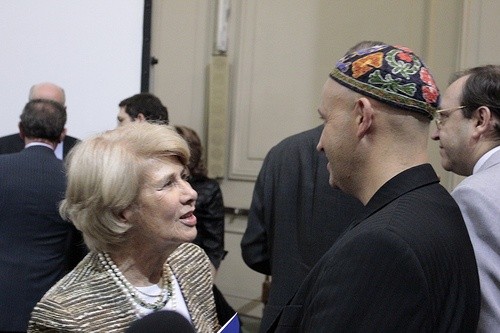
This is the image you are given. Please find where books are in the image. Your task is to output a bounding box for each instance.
[213,312,241,333]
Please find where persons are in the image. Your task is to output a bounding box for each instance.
[1,82,83,162]
[428,64,500,333]
[260,45,482,333]
[239,40,392,325]
[25,124,222,332]
[115,92,170,129]
[169,126,237,326]
[0,98,80,333]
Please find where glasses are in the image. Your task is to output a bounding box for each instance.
[434,105,472,130]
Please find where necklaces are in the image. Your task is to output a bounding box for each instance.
[96,248,173,318]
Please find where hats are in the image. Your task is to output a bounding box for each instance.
[329,41,440,120]
